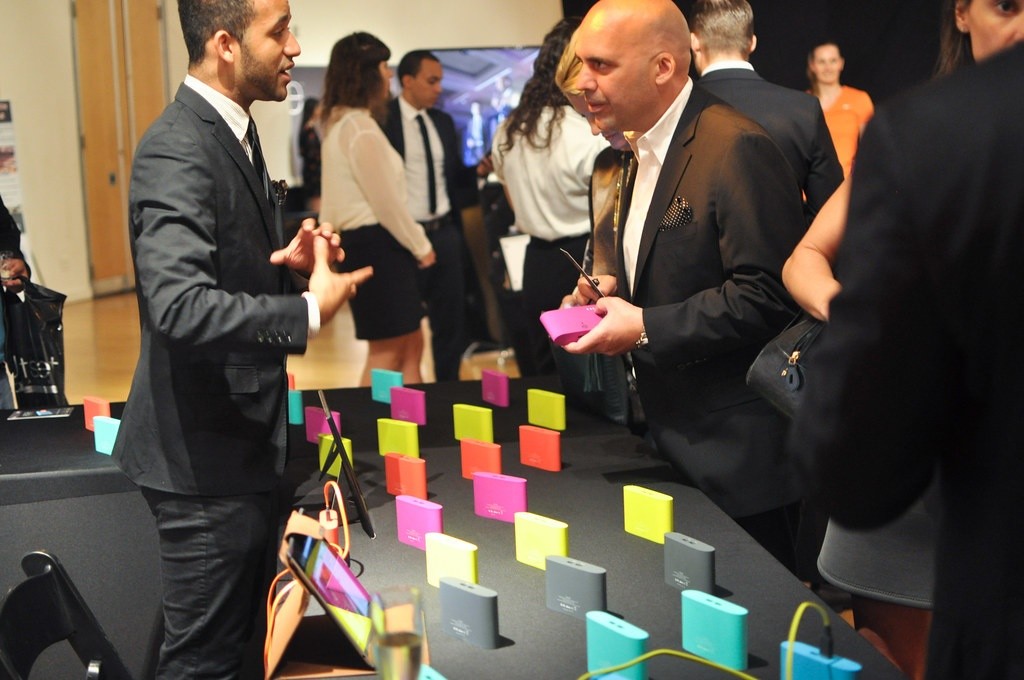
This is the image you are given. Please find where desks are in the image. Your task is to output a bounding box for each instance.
[277,378,904,680]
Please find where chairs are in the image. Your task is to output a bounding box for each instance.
[0,552,132,680]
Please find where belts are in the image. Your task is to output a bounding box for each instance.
[425,212,454,232]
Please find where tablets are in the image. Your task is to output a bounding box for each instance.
[287,507,388,669]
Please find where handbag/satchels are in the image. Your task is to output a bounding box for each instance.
[745,316,829,417]
[1,274,71,409]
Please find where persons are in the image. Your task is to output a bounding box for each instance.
[490,0,1024,680]
[0,192,32,367]
[112,1,486,680]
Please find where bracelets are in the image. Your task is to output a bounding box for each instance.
[636,331,648,349]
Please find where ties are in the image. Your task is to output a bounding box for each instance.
[245,117,265,192]
[416,113,436,214]
